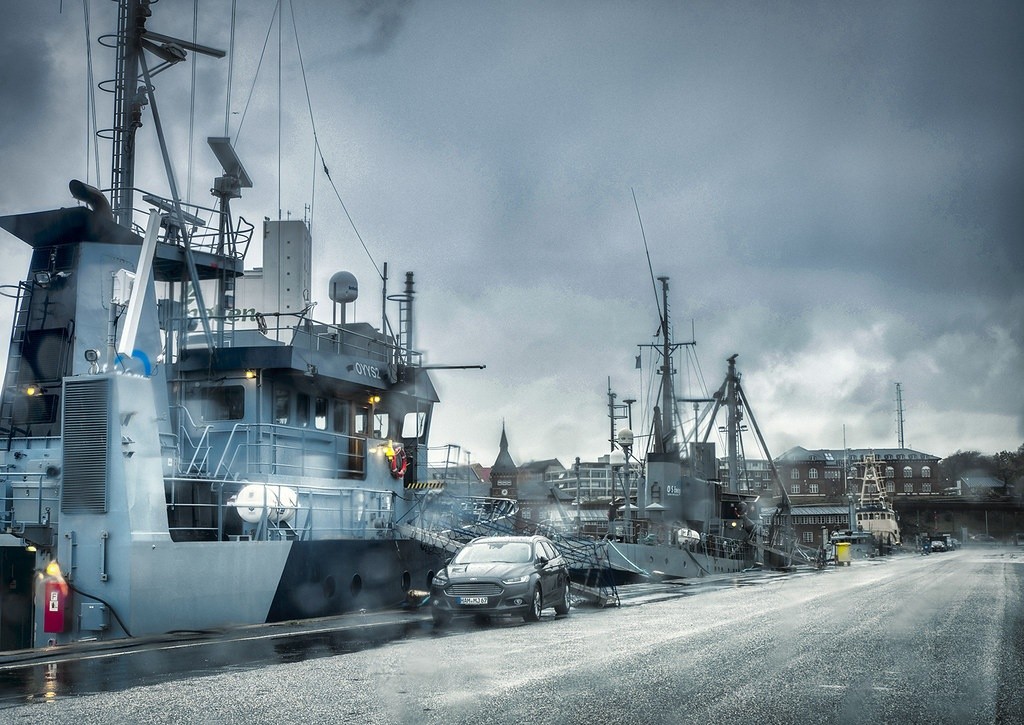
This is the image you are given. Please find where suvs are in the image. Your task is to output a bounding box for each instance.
[430,535,572,627]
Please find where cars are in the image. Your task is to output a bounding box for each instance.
[927,533,995,552]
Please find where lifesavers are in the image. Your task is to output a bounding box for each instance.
[390,447,408,478]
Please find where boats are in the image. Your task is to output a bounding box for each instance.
[821,523,897,559]
[852,449,903,550]
[0,3,525,649]
[529,275,798,587]
